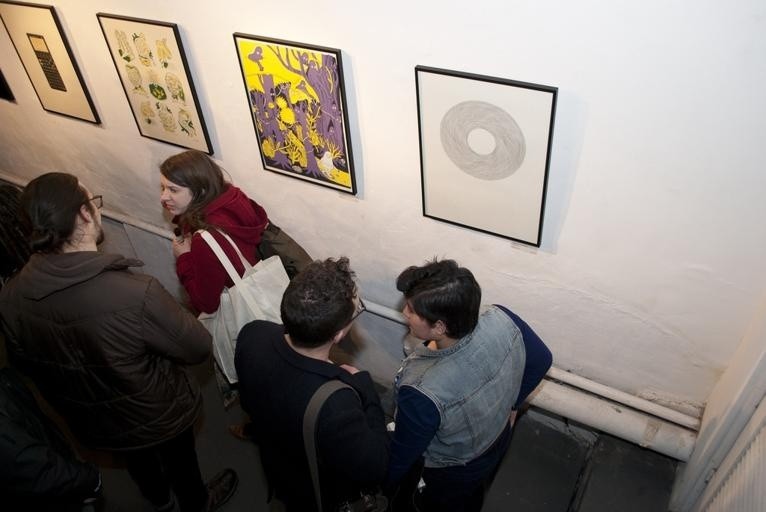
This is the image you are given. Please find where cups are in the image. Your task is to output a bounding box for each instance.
[81,194,103,210]
[339,297,367,332]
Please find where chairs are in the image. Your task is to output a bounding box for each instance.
[188,465,240,511]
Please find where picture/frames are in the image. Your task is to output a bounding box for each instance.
[0,1,101,125]
[96,12,214,157]
[234,31,357,197]
[414,65,557,246]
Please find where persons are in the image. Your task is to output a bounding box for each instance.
[382,257,550,512]
[156,148,270,445]
[0,180,125,470]
[1,354,104,512]
[0,170,239,512]
[232,253,391,512]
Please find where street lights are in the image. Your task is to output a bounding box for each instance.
[193,220,313,387]
[329,493,388,512]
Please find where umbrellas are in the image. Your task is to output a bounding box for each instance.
[228,424,256,443]
